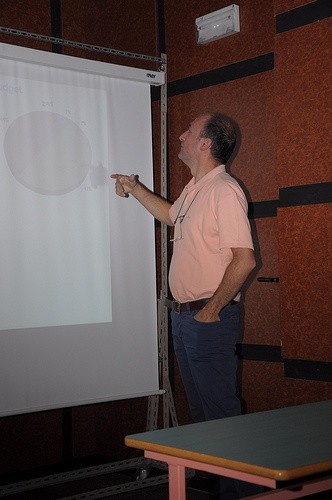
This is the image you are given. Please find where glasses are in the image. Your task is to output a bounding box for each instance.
[169,216,184,241]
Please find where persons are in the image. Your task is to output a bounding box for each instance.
[110,113,258,500]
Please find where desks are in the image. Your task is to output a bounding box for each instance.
[121,399,332,500]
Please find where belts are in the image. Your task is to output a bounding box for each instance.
[172,298,240,313]
